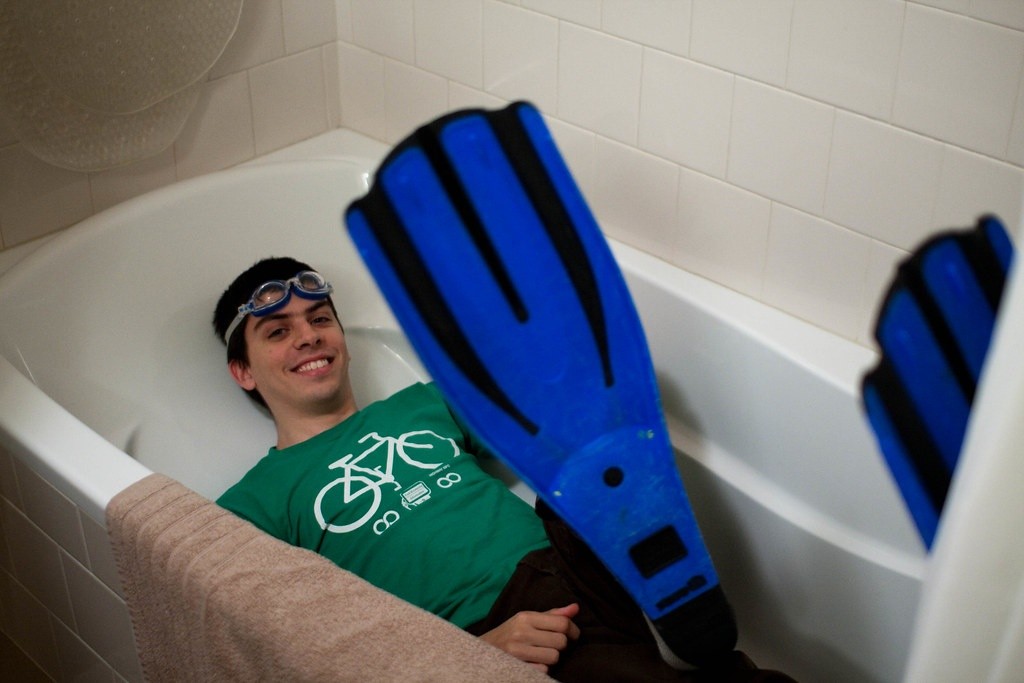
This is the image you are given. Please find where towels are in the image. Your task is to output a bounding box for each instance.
[106,473,558,682]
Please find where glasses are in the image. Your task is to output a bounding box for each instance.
[225,270,331,346]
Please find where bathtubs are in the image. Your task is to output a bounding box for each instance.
[0,127,934,682]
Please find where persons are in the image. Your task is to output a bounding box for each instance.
[212,257,799,683]
